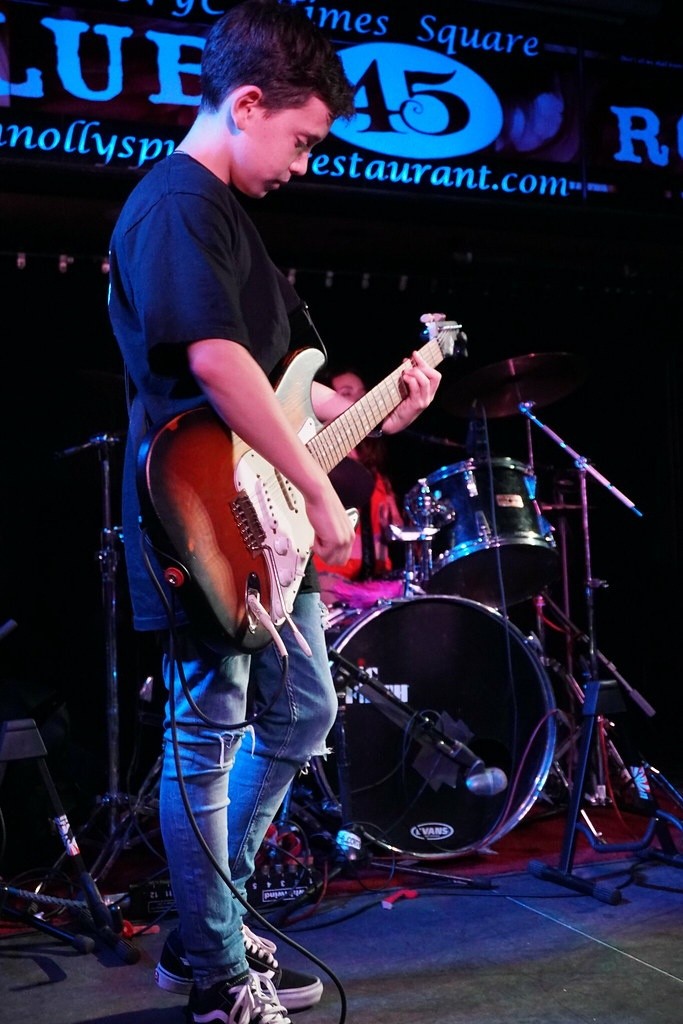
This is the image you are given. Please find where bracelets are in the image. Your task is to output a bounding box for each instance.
[373,421,383,438]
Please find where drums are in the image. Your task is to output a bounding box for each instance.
[292,588,559,863]
[403,452,563,610]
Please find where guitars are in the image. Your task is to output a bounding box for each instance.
[134,308,464,662]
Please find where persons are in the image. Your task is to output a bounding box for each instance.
[106,0,442,1024]
[312,367,435,612]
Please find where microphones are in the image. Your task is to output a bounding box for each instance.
[445,739,508,797]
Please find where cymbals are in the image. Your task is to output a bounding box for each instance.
[538,501,586,513]
[442,345,590,418]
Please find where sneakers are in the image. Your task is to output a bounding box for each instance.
[153,916,324,1009]
[187,966,293,1024]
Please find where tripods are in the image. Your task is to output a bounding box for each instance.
[262,648,499,936]
[21,458,160,920]
[512,384,644,827]
